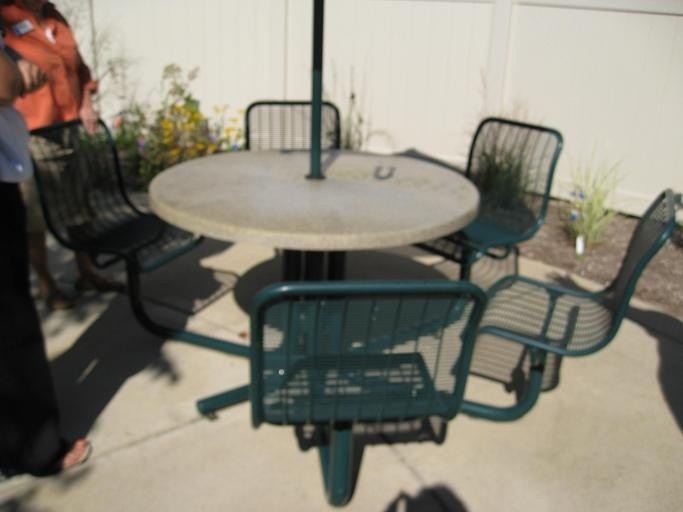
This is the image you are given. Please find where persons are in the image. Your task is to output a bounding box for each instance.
[0,27,92,475]
[0,0,127,310]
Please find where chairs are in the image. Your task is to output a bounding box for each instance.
[452,117,564,281]
[457,188,676,423]
[248,280,487,507]
[243,99,341,150]
[26,117,203,324]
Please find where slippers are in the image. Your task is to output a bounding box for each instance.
[43,285,70,311]
[79,267,110,287]
[55,435,92,470]
